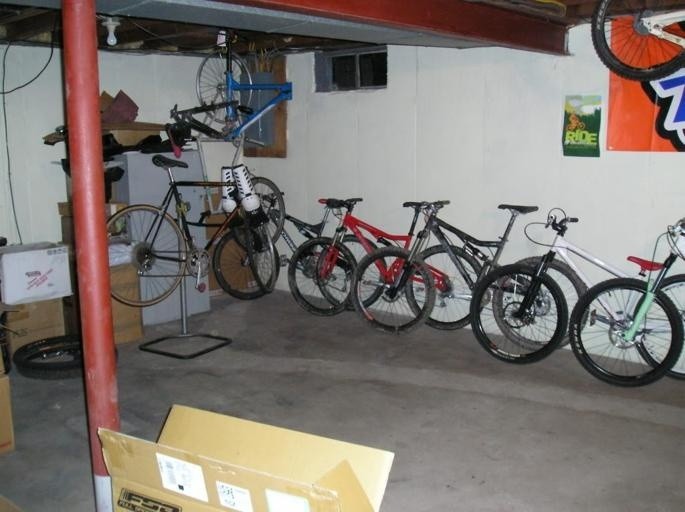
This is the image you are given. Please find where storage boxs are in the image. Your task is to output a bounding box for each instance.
[204,194,260,297]
[0,201,145,452]
